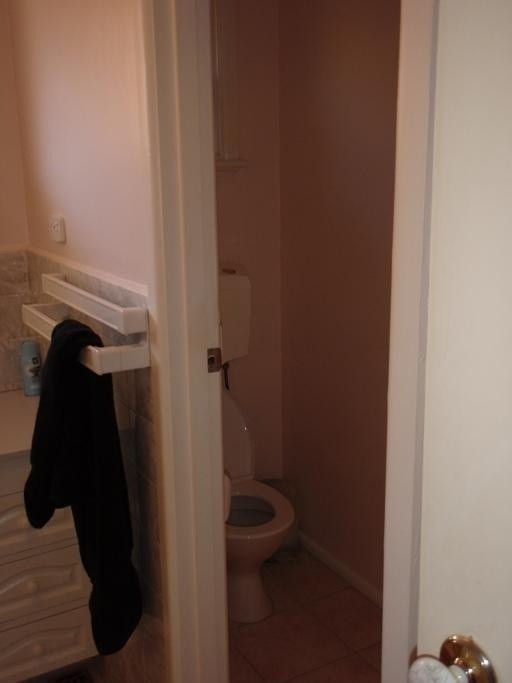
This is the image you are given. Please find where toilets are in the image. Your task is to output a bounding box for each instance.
[218,272,295,625]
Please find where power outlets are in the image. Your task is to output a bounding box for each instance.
[47,216,65,242]
